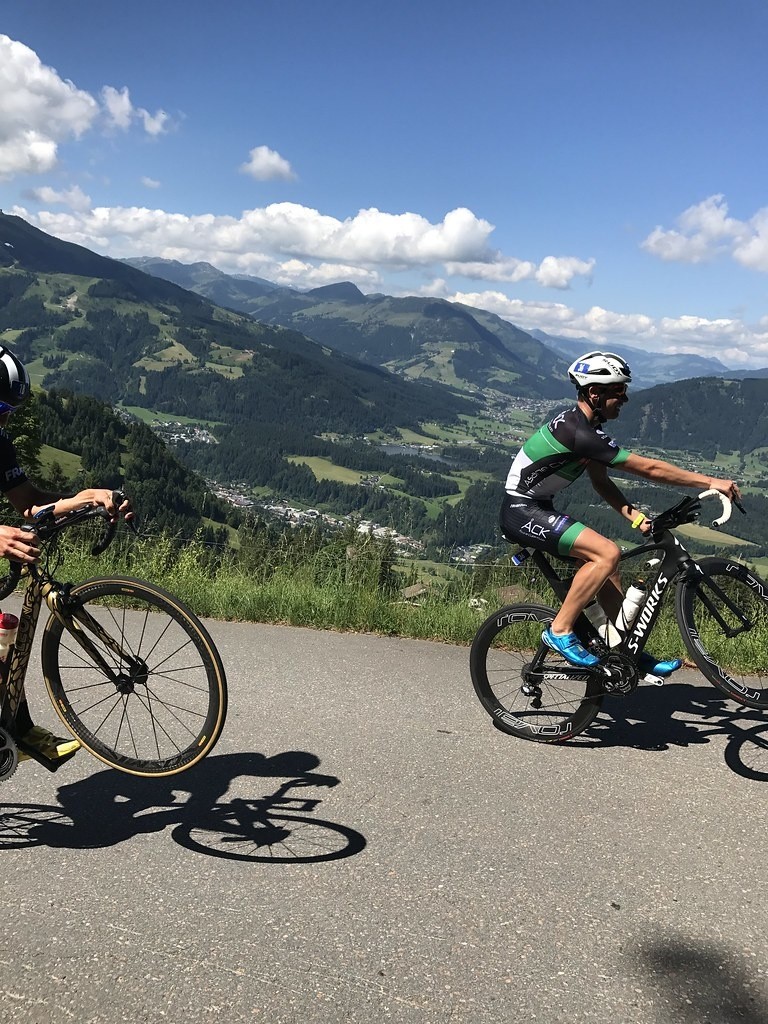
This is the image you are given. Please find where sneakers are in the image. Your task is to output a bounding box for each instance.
[636,652,682,676]
[541,625,600,666]
[13,725,81,763]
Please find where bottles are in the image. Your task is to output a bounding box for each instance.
[615,582,649,632]
[584,600,622,649]
[0,613,19,663]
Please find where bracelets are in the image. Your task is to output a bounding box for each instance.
[631,513,646,529]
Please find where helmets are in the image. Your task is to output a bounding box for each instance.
[0,345,30,406]
[567,350,632,389]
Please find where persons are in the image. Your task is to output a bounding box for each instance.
[0,348,135,763]
[497,352,742,677]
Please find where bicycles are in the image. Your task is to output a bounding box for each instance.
[0,488,230,786]
[466,485,767,745]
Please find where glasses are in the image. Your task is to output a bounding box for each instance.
[594,384,628,398]
[0,401,19,415]
